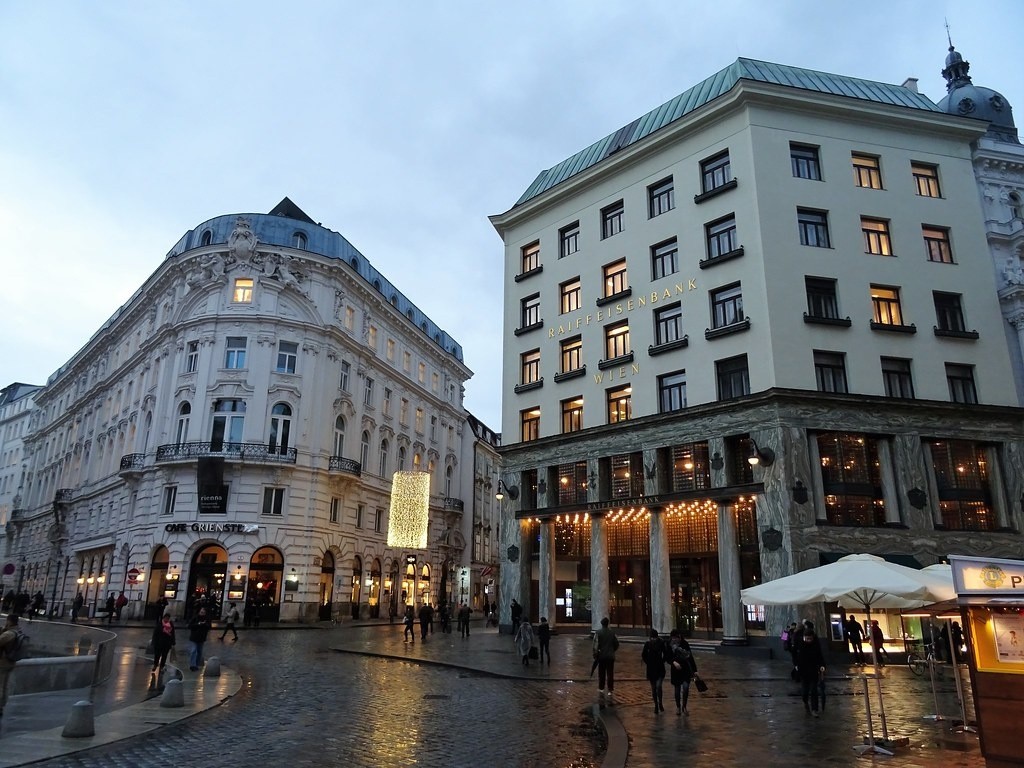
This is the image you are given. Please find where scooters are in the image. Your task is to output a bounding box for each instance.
[485,610,500,628]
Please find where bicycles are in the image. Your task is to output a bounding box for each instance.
[907,641,938,675]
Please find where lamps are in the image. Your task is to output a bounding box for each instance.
[76,572,85,585]
[235,563,242,580]
[365,573,374,586]
[495,478,519,502]
[97,570,106,583]
[401,576,408,589]
[291,567,298,583]
[165,564,177,580]
[86,572,95,585]
[418,577,425,588]
[384,575,392,588]
[744,432,776,472]
[135,566,146,582]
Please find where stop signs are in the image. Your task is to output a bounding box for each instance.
[128,568,139,580]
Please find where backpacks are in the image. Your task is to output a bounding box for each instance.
[233,610,239,621]
[5,629,29,662]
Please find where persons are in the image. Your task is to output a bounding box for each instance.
[150,595,176,673]
[514,616,535,665]
[188,607,212,672]
[403,601,439,643]
[537,616,551,664]
[510,598,522,633]
[592,616,619,696]
[664,629,698,717]
[218,601,239,642]
[440,600,474,638]
[70,591,128,624]
[845,615,868,665]
[484,600,499,628]
[871,620,886,667]
[0,590,45,707]
[641,628,673,714]
[783,620,827,719]
[928,620,966,663]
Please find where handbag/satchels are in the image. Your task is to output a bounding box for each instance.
[529,644,538,659]
[694,675,708,692]
[457,624,461,631]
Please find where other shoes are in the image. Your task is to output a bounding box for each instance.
[232,637,238,640]
[607,692,612,695]
[805,703,810,713]
[462,636,464,638]
[152,665,157,671]
[410,641,414,643]
[660,705,664,712]
[654,707,658,713]
[676,710,680,715]
[190,667,197,671]
[811,711,818,717]
[160,669,164,673]
[218,637,223,640]
[404,640,407,642]
[597,689,604,692]
[683,708,688,714]
[467,634,470,637]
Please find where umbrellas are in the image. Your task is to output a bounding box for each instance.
[739,554,960,738]
[819,667,826,714]
[836,558,968,727]
[590,660,599,678]
